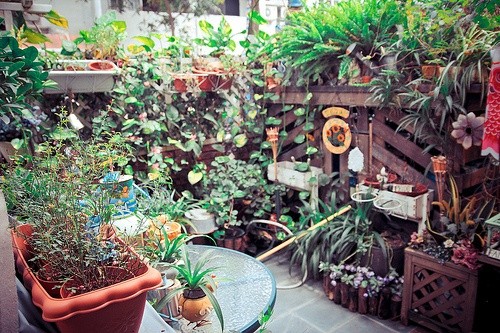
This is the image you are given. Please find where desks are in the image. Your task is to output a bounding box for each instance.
[167,245,277,333]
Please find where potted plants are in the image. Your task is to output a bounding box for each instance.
[12,0,500,333]
